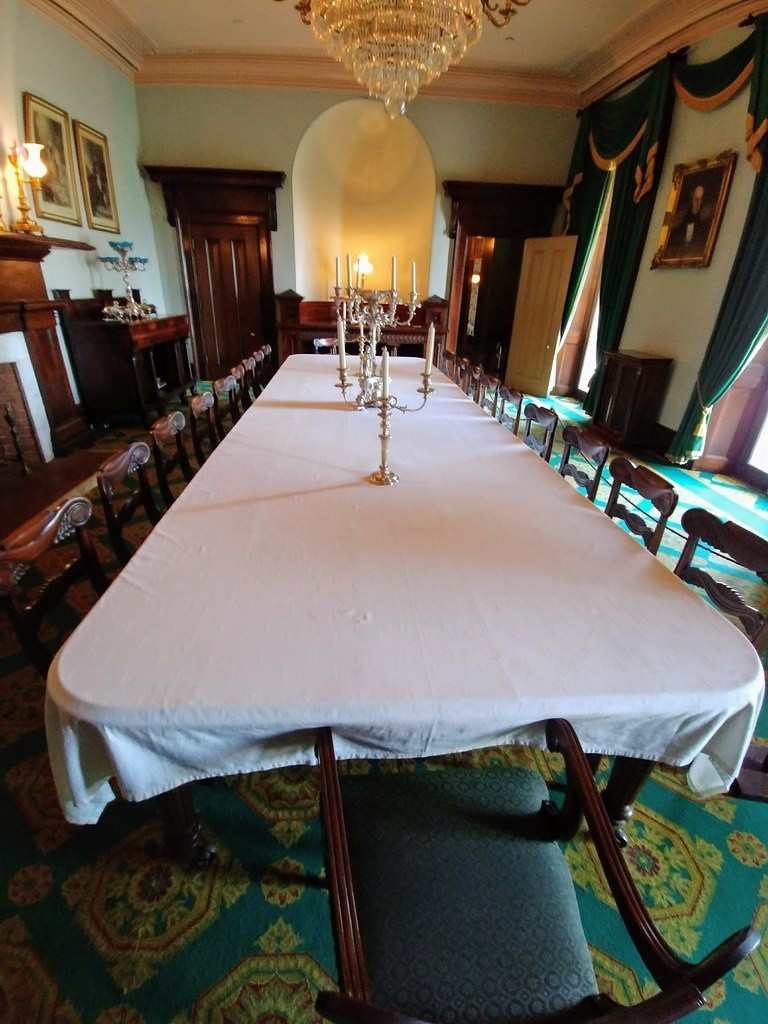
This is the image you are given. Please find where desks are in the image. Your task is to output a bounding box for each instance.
[38,350,765,822]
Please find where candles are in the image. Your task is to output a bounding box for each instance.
[391,256,397,290]
[336,315,346,369]
[336,256,340,287]
[347,253,352,288]
[357,259,361,287]
[382,345,389,400]
[424,322,435,376]
[360,322,363,336]
[412,261,416,292]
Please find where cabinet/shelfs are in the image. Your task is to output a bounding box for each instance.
[55,287,199,436]
[589,349,674,449]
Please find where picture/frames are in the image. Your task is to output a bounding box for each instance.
[650,150,735,270]
[24,91,82,226]
[73,119,121,233]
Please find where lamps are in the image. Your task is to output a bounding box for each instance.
[471,270,480,283]
[298,0,530,121]
[8,139,48,234]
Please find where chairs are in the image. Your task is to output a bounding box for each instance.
[437,349,768,658]
[0,342,273,683]
[315,720,761,1024]
